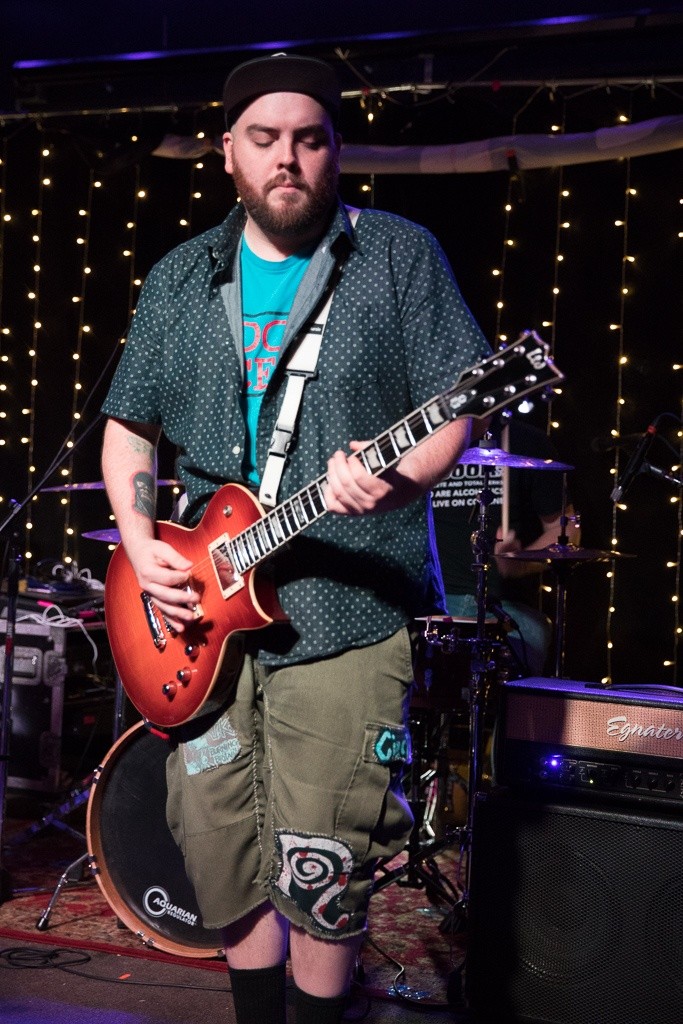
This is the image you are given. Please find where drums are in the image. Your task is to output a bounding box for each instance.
[408,614,505,713]
[87,719,226,958]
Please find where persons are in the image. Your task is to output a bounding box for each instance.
[98,46,581,1024]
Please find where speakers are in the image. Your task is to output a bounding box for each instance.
[463,788,683,1024]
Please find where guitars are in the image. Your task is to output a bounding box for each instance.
[106,330,566,728]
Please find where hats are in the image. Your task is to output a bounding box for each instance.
[224,52,339,133]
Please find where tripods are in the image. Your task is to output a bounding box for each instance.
[372,711,463,914]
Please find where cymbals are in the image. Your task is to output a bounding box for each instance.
[39,478,183,492]
[80,529,121,544]
[457,444,575,472]
[491,543,638,560]
